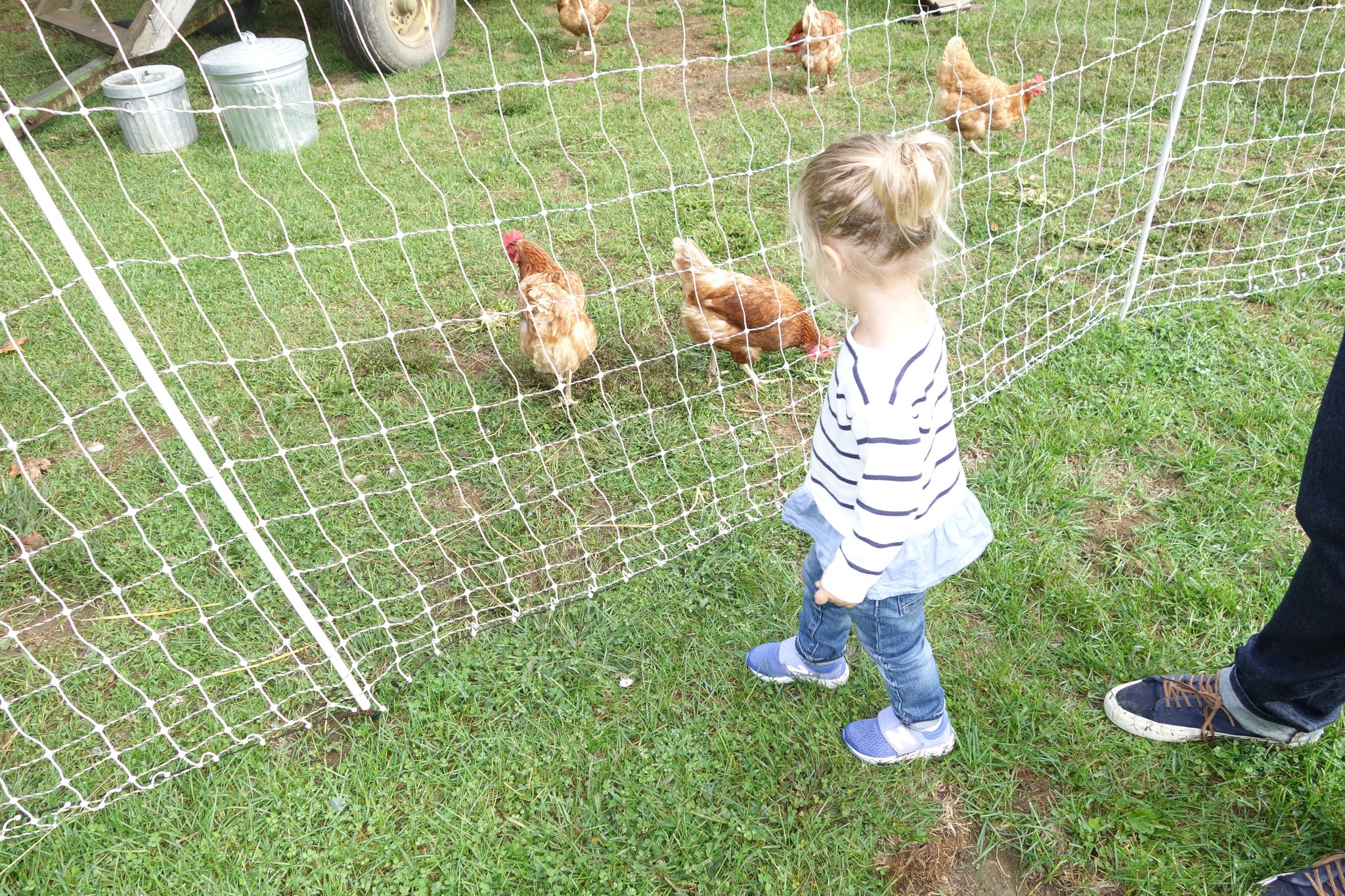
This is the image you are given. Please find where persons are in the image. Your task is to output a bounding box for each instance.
[745,133,993,764]
[1098,324,1345,896]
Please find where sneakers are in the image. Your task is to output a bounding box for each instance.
[1103,670,1324,749]
[1243,851,1345,896]
[746,635,849,691]
[842,705,957,766]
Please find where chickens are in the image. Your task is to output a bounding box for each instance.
[499,226,601,412]
[672,236,836,389]
[935,36,1048,159]
[784,0,845,92]
[554,1,610,57]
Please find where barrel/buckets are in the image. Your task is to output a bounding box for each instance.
[101,64,200,156]
[201,30,320,154]
